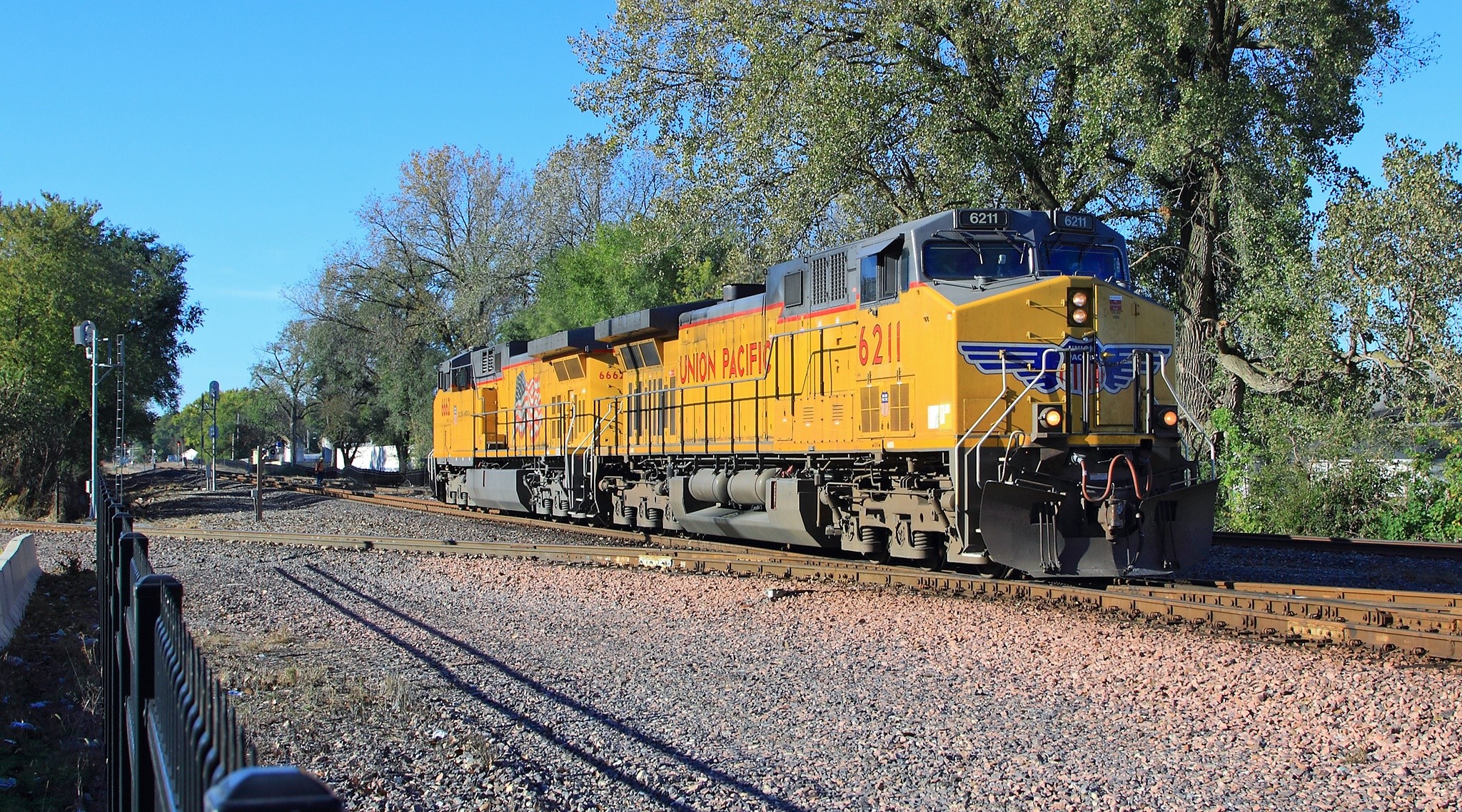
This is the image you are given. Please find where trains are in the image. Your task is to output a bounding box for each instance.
[420,200,1222,588]
